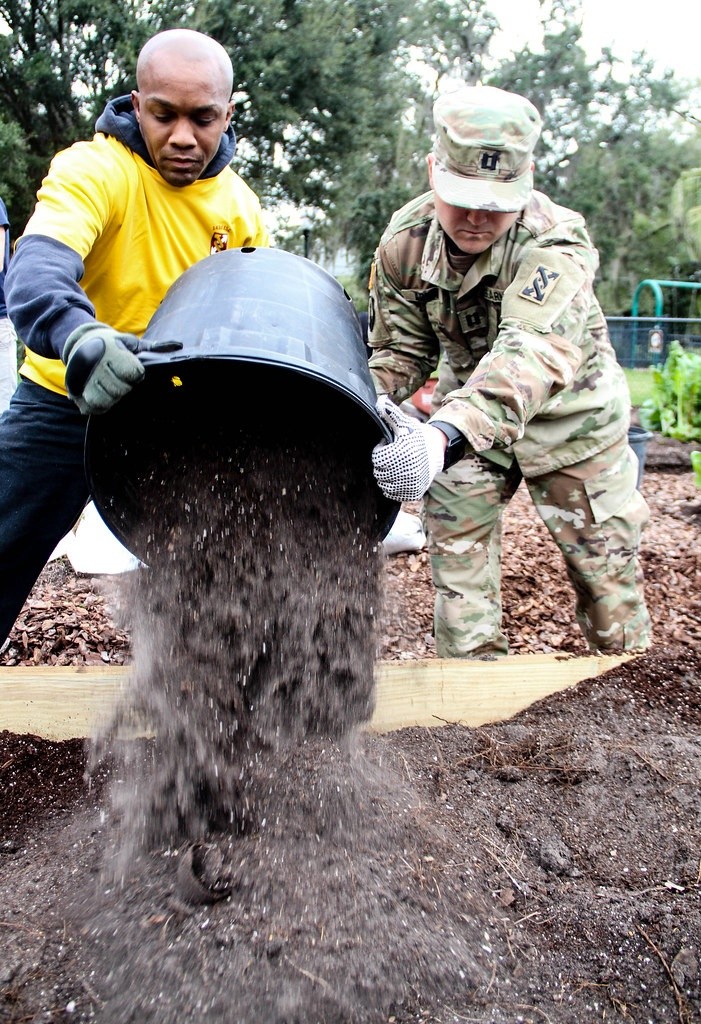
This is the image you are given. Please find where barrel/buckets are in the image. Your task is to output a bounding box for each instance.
[627,426,649,493]
[82,246,401,572]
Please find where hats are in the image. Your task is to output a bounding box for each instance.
[432,86,540,211]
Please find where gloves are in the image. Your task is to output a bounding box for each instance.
[371,395,446,501]
[62,322,184,416]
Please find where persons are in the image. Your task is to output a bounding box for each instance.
[0,28,270,649]
[366,86,653,661]
[0,197,18,414]
